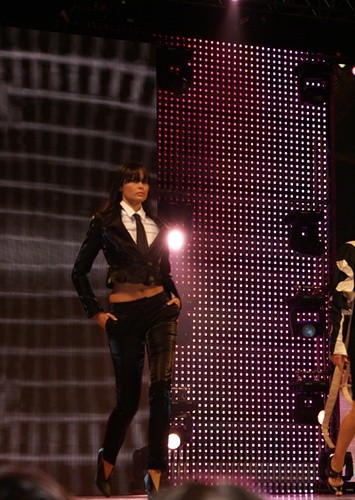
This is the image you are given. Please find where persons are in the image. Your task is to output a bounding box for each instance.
[325,240,355,496]
[71,160,181,500]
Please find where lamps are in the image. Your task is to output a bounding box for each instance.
[166,387,197,450]
[295,60,330,106]
[287,286,327,339]
[282,210,324,257]
[153,45,194,94]
[292,378,330,425]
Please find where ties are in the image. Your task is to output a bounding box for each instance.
[133,214,148,251]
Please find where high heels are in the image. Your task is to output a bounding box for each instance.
[145,471,162,499]
[96,449,113,497]
[328,456,344,496]
